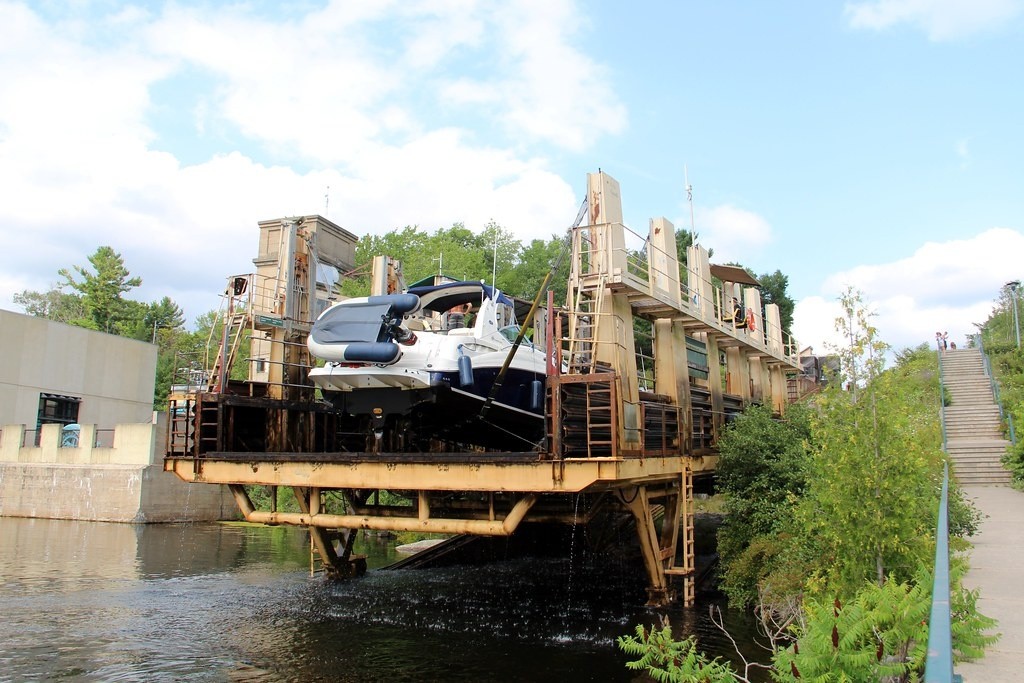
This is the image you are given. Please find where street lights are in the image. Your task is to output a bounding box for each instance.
[1007,282,1022,351]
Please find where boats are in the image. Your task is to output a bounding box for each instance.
[304,277,578,449]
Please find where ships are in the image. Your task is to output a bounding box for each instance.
[164,169,805,613]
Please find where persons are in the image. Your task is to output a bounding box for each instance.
[732,297,741,313]
[935,331,957,351]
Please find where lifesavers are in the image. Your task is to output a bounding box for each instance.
[747,308,755,332]
